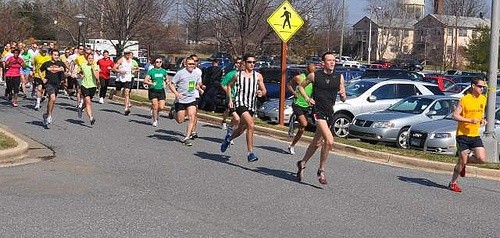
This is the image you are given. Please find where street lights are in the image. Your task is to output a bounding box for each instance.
[73,13,88,49]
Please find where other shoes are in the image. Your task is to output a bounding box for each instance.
[182,138,192,146]
[222,120,227,130]
[157,112,159,120]
[3,85,131,129]
[189,135,194,140]
[169,106,175,119]
[192,131,198,138]
[153,120,158,127]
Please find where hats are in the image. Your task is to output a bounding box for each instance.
[124,49,130,53]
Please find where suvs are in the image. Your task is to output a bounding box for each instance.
[327,77,450,138]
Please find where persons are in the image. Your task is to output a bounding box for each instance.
[200,53,266,162]
[287,63,315,155]
[169,55,206,146]
[0,38,114,129]
[110,50,140,115]
[145,55,170,127]
[297,51,346,185]
[448,77,487,192]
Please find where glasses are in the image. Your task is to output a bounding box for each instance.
[245,60,256,64]
[475,84,486,89]
[156,61,163,64]
[194,60,199,62]
[187,63,195,66]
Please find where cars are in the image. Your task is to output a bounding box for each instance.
[348,94,470,149]
[108,65,148,89]
[197,51,500,122]
[407,98,500,162]
[257,94,300,125]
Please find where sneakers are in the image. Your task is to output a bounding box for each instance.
[247,153,258,161]
[448,183,462,192]
[288,122,295,136]
[227,126,233,137]
[297,161,306,181]
[289,146,295,155]
[221,134,230,152]
[317,169,327,184]
[460,165,465,176]
[231,139,235,145]
